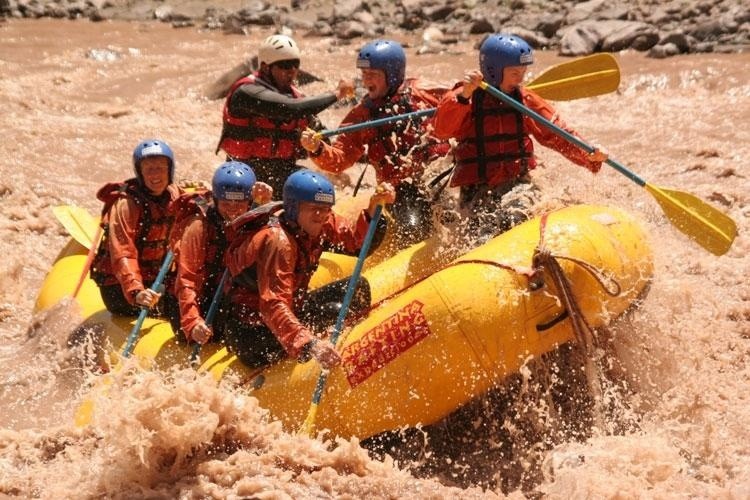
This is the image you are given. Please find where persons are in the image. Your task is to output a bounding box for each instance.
[433,32,609,245]
[167,160,273,348]
[224,169,397,370]
[90,139,213,319]
[299,39,459,249]
[214,34,357,201]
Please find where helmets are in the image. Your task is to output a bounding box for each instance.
[212,161,256,203]
[479,34,533,88]
[132,139,174,185]
[258,35,300,71]
[283,168,335,223]
[356,39,406,95]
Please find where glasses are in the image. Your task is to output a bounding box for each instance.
[274,59,300,70]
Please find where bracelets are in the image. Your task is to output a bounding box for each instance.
[131,290,139,303]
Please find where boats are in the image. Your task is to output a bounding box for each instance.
[28,191,654,454]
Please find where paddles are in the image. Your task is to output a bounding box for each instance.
[313,52,620,139]
[476,78,737,257]
[51,204,106,254]
